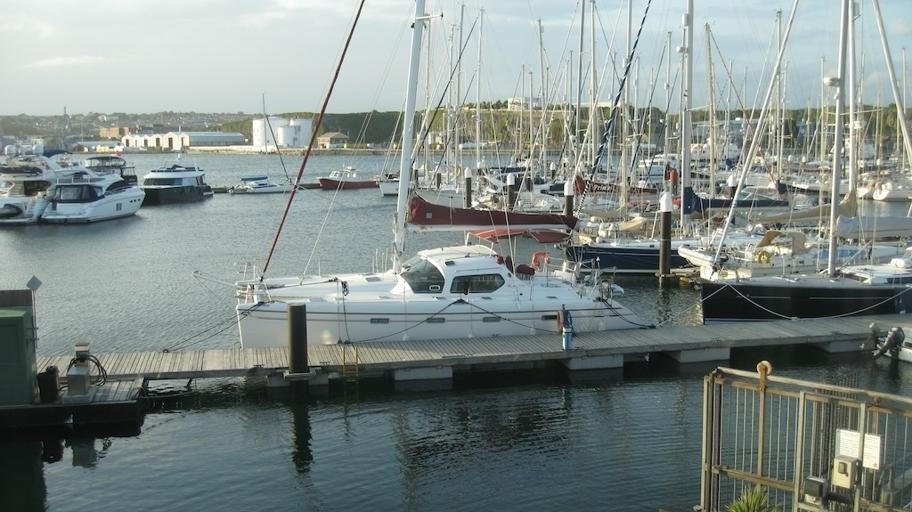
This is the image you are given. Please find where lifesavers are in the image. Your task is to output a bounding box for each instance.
[757,250,770,263]
[455,167,461,176]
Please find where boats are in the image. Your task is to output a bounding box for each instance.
[0,143,215,226]
